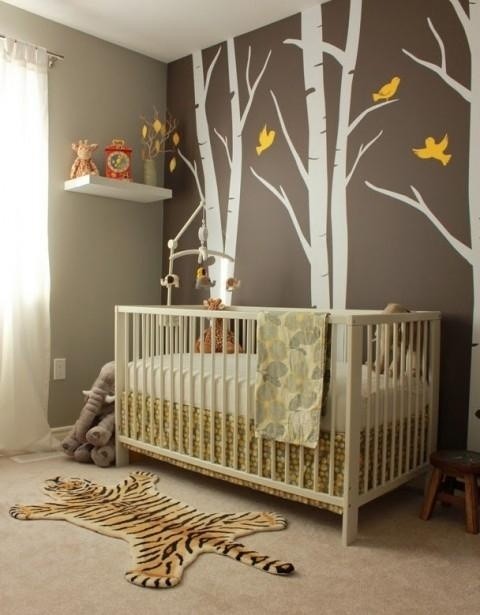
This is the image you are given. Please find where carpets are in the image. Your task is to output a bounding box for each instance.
[5,473,298,591]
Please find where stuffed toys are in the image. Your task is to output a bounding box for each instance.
[70,140,100,179]
[160,273,179,288]
[226,278,241,292]
[195,268,216,290]
[365,303,423,378]
[60,360,116,467]
[194,298,244,354]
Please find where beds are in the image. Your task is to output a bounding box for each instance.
[112,295,445,552]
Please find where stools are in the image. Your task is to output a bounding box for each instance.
[418,446,479,536]
